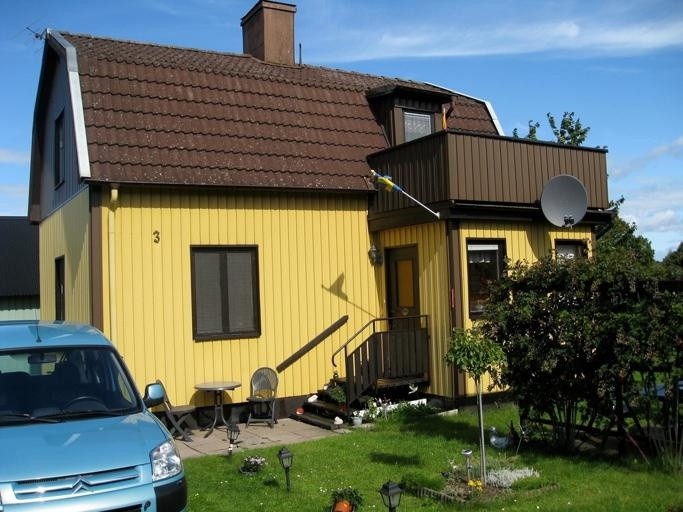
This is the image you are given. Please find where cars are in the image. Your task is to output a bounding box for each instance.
[0,317,189,512]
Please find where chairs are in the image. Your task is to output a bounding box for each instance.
[2,372,32,413]
[246,366,278,429]
[155,379,196,443]
[48,375,104,405]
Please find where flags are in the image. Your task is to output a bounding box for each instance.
[370,172,404,195]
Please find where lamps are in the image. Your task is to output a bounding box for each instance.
[368,240,383,265]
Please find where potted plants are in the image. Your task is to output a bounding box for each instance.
[328,385,348,411]
[332,486,363,512]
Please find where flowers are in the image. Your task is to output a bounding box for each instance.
[352,410,365,417]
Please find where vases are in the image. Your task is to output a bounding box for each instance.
[353,417,362,426]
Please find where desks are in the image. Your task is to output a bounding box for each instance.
[194,381,241,438]
